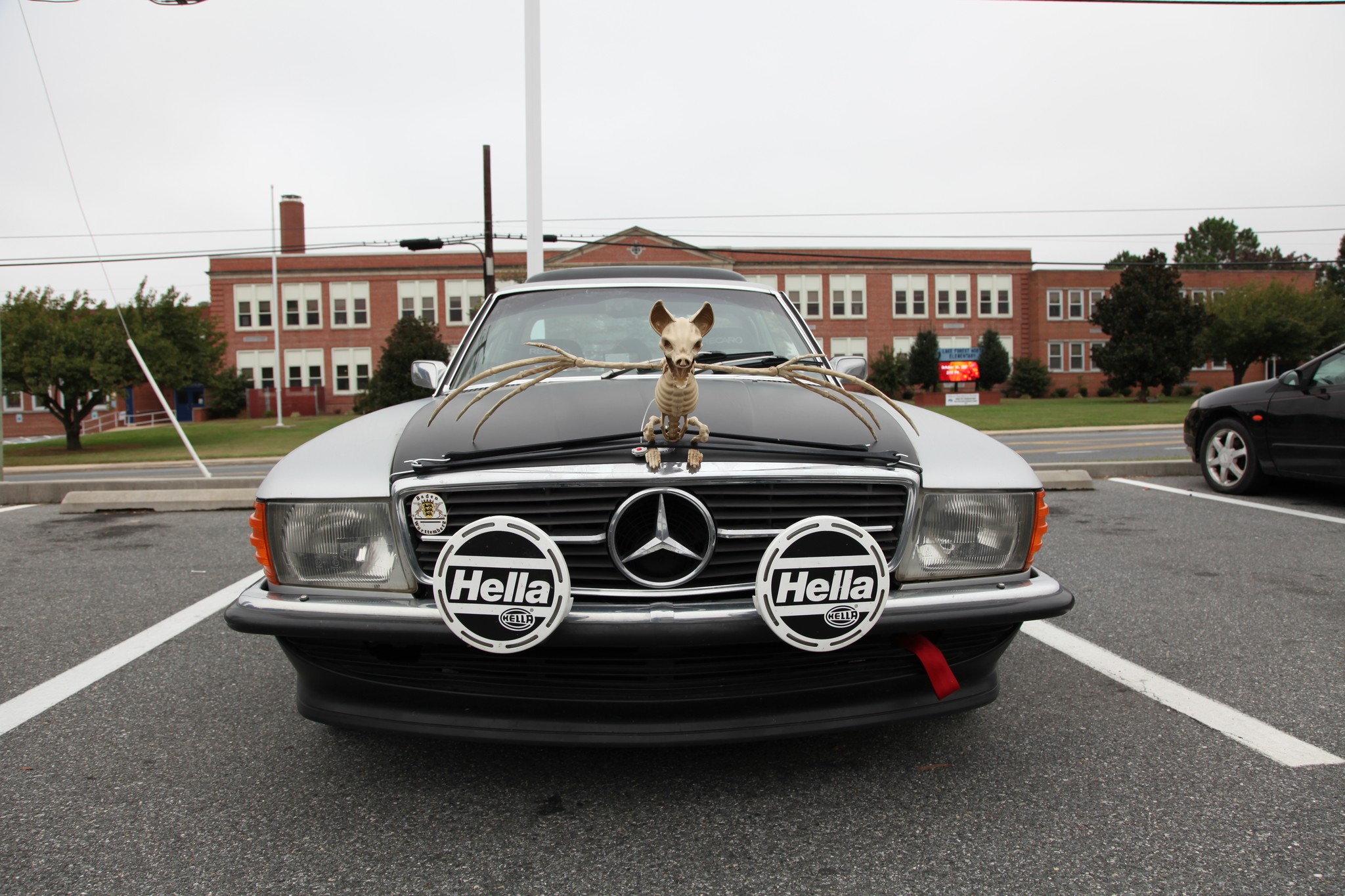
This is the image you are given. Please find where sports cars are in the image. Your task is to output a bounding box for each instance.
[225,264,1077,751]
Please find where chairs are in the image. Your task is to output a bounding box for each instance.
[702,328,754,351]
[518,338,584,369]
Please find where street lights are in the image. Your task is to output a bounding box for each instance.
[396,144,495,300]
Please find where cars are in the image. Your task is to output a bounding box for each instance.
[1182,343,1345,496]
